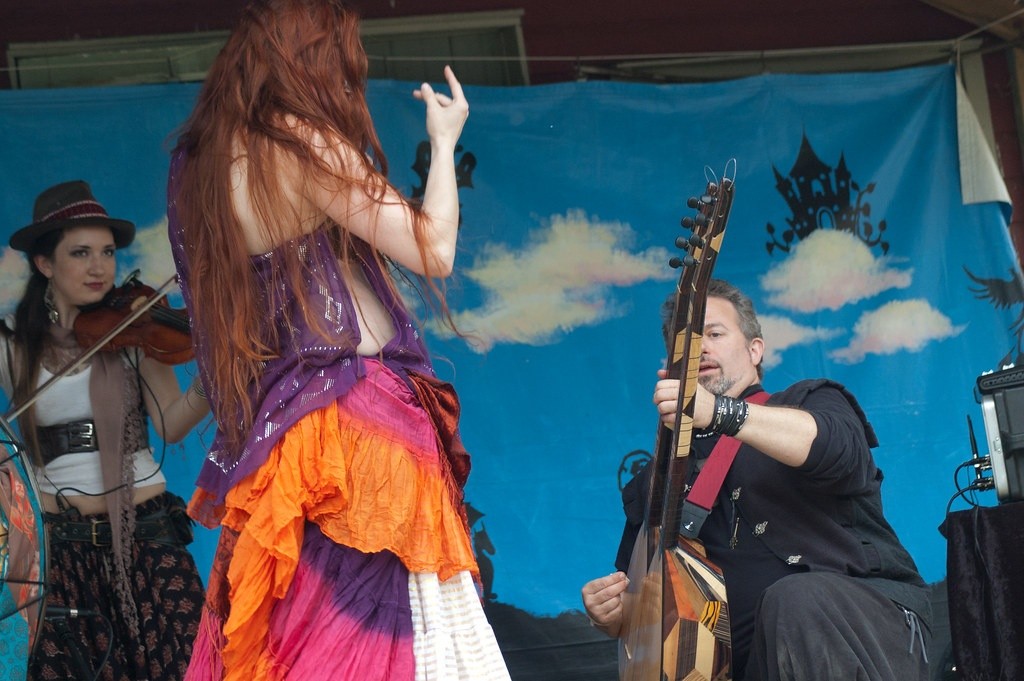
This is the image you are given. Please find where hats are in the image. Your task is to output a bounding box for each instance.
[9,180,136,251]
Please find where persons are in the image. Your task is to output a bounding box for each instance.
[581,278,934,681]
[0,179,212,681]
[165,1,517,681]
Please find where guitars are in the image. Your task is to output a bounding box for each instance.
[614,157,742,681]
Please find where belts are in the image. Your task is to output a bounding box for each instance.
[40,514,186,552]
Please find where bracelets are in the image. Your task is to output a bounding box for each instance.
[586,614,613,628]
[192,380,210,399]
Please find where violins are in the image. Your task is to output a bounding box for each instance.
[73,273,191,362]
